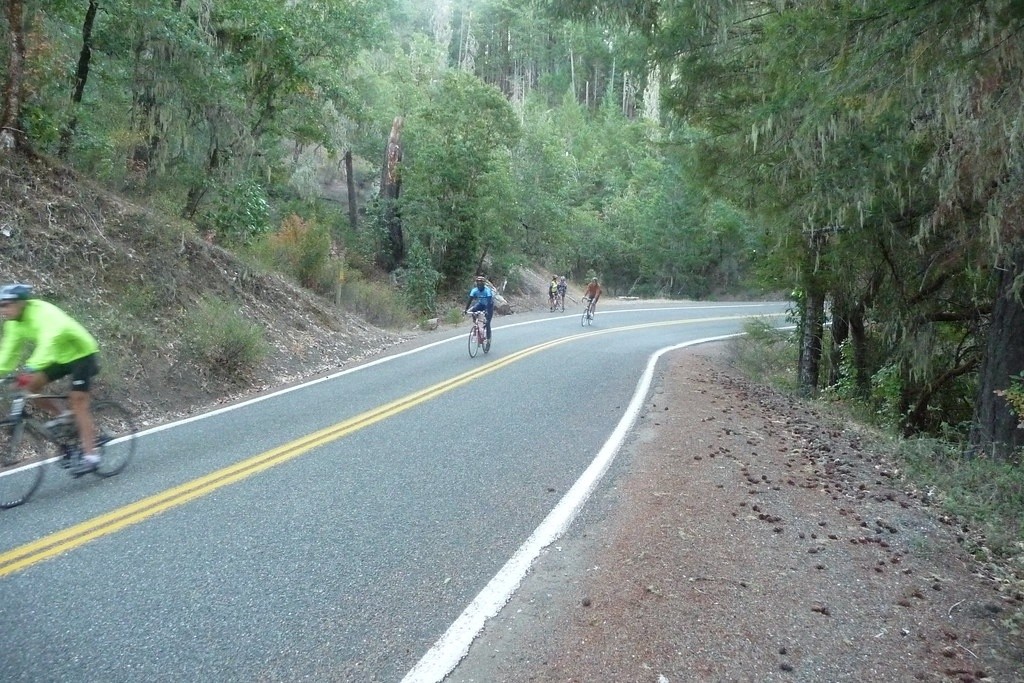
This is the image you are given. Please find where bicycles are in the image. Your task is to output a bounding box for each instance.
[580,296,594,327]
[462,311,492,358]
[547,291,564,313]
[0,365,138,511]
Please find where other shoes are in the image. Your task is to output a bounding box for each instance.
[485,343,490,352]
[45,412,76,429]
[473,330,476,336]
[67,459,99,475]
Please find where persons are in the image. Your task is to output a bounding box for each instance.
[546,275,602,318]
[0,285,103,473]
[463,276,493,350]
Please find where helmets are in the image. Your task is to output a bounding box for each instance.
[560,276,565,281]
[553,275,558,279]
[0,285,34,300]
[591,277,598,283]
[476,276,486,285]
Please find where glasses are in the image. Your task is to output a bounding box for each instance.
[0,301,16,308]
